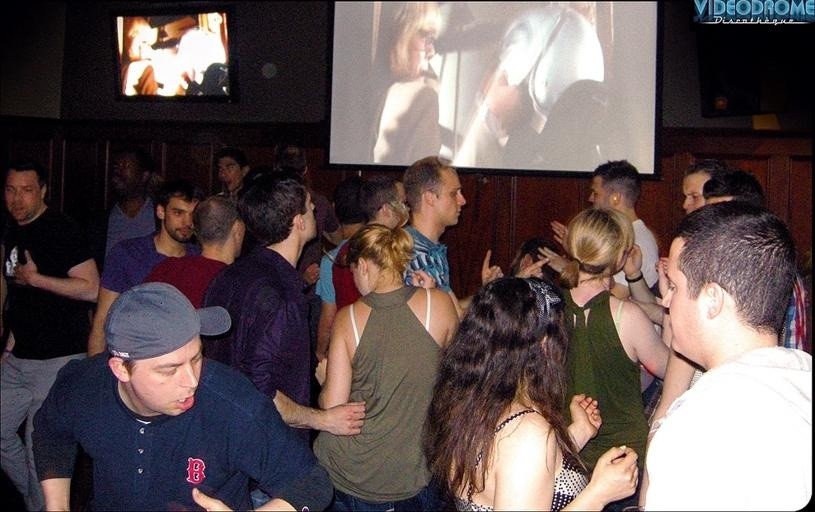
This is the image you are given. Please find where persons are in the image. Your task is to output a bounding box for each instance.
[450,0,599,171]
[121,12,227,97]
[373,1,443,165]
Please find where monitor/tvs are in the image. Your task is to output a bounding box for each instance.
[323,0,663,182]
[111,6,241,104]
[696,25,815,117]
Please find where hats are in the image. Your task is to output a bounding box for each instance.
[103,280,233,361]
